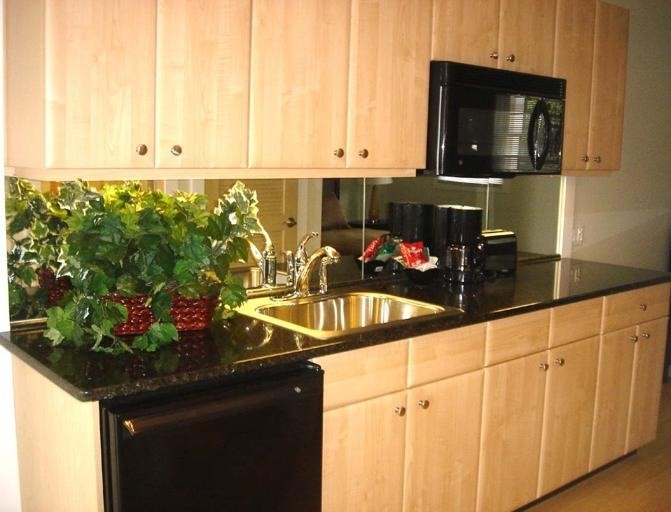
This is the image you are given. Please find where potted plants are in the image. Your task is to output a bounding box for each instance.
[42,181,273,354]
[5,175,91,323]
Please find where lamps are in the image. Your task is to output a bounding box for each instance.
[357,177,393,223]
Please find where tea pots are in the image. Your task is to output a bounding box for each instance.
[439,287,483,322]
[438,233,488,284]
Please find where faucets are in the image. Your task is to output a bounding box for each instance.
[245,227,278,287]
[292,231,342,296]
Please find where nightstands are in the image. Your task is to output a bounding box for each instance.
[347,219,391,230]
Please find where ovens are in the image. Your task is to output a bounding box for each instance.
[102,359,323,511]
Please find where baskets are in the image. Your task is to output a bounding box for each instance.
[36,266,221,337]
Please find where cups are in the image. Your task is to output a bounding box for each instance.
[434,201,484,242]
[387,199,434,245]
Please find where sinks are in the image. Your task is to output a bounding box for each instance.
[217,290,466,341]
[218,266,297,304]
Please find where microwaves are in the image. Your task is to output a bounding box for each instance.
[424,61,567,176]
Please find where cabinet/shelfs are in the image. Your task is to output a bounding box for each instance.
[475,295,604,511]
[250,1,434,179]
[4,2,249,180]
[431,1,557,78]
[553,0,630,176]
[588,281,670,474]
[308,322,487,512]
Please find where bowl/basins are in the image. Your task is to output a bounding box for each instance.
[397,259,442,285]
[355,252,397,278]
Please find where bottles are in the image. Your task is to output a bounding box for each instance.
[249,266,264,288]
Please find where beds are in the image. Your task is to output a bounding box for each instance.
[321,192,392,255]
